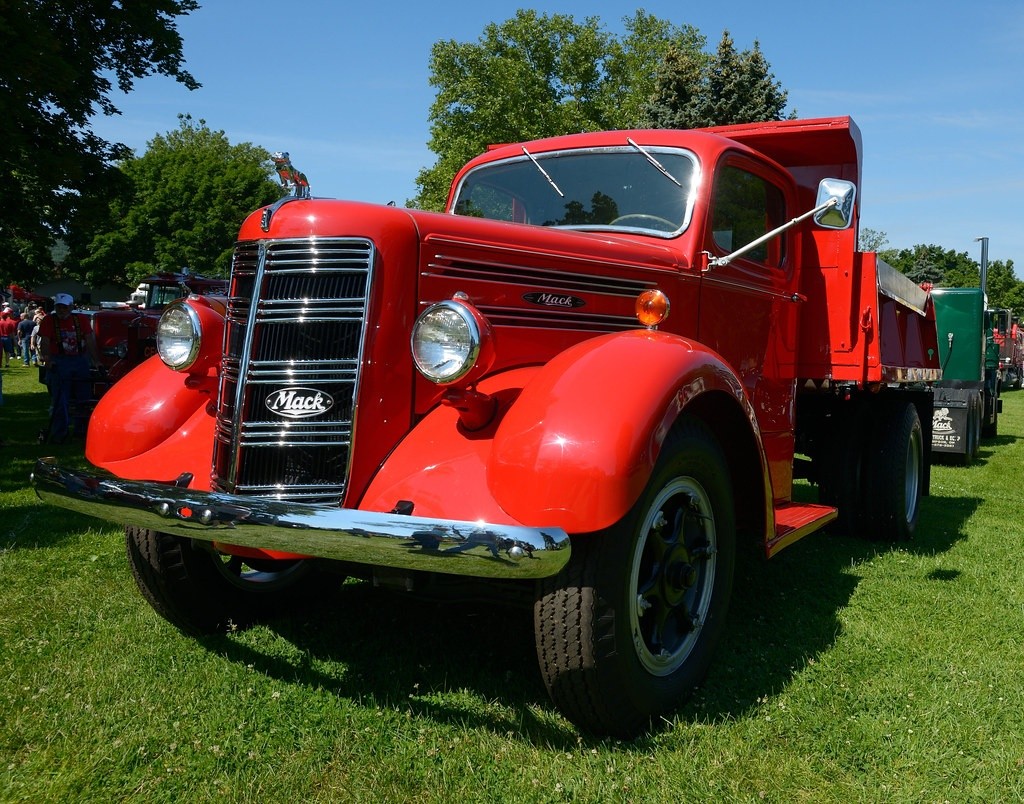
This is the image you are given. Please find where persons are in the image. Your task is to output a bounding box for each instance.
[36,294,94,441]
[0,300,47,368]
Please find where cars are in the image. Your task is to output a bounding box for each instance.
[71,266,231,400]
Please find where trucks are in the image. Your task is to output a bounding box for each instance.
[847,234,1024,468]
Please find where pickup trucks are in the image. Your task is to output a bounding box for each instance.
[29,113,945,739]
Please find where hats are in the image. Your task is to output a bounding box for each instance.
[2,302,9,306]
[0,312,7,318]
[54,293,74,305]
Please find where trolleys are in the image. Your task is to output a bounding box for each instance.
[36,356,114,447]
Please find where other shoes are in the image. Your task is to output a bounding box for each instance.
[17,356,21,360]
[5,364,9,368]
[22,365,31,368]
[9,356,14,359]
[33,362,38,366]
